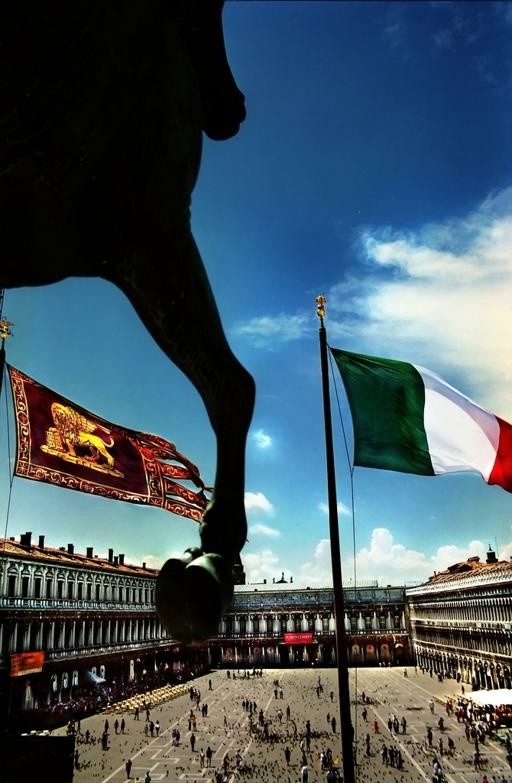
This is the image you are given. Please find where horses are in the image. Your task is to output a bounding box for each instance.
[0,1,262,663]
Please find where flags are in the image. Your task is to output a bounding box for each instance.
[329,346,510,489]
[4,363,218,521]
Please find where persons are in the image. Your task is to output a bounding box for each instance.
[44,667,511,782]
